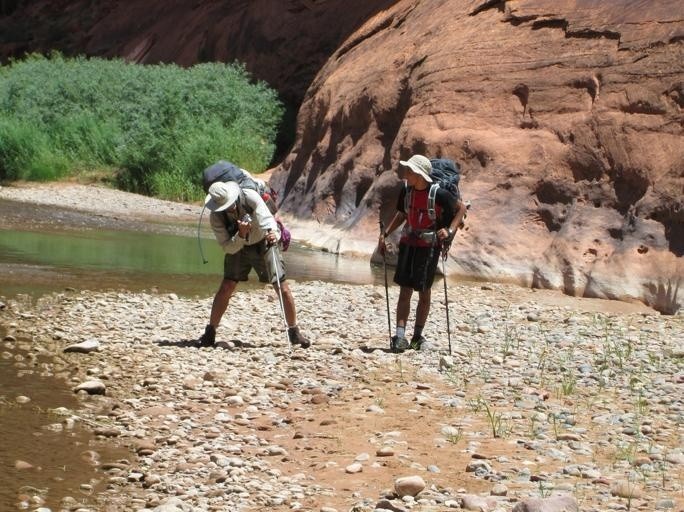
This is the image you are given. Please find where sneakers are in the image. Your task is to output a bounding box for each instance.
[288,326,310,347]
[193,326,217,344]
[409,336,425,350]
[391,336,410,353]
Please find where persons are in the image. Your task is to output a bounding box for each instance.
[376,154,469,353]
[194,179,313,348]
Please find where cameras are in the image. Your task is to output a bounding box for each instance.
[241,213,253,226]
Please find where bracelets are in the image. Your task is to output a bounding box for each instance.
[381,231,389,239]
[447,228,454,235]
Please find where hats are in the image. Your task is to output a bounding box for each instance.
[204,180,240,212]
[399,154,433,183]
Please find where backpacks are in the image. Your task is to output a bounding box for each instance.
[402,158,463,232]
[204,160,279,216]
